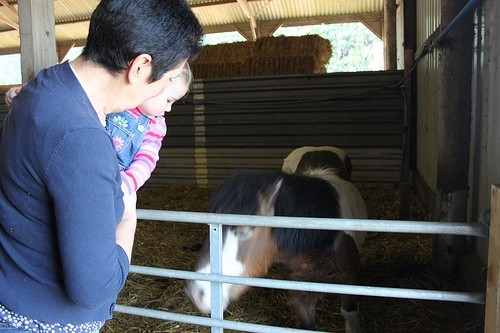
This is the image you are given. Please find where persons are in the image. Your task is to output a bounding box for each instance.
[5,60,192,195]
[0,0,202,333]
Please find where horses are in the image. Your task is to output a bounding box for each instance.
[184,145,369,333]
